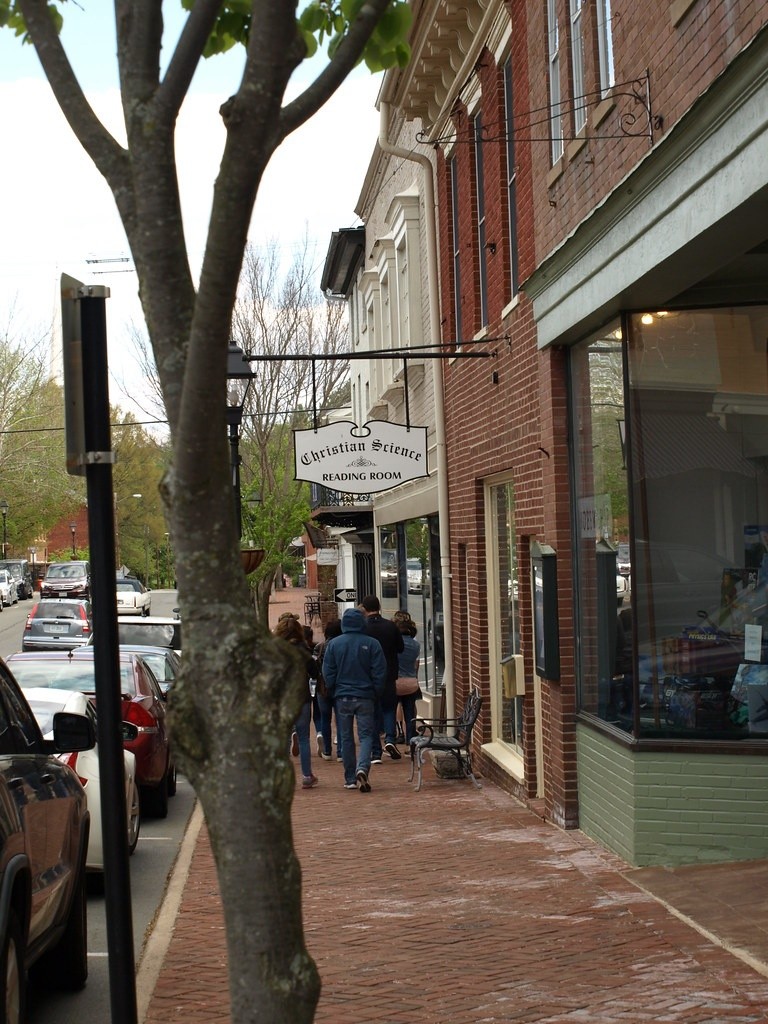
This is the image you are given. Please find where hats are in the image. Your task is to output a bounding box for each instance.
[278,612,300,624]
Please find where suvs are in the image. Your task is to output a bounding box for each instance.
[0,656,94,1024]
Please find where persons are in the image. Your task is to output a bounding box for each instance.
[322,608,388,793]
[394,617,420,745]
[274,618,319,789]
[278,612,300,755]
[314,619,343,761]
[390,611,420,744]
[360,596,405,763]
[302,625,322,757]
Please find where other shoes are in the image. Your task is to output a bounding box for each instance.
[397,733,405,745]
[316,732,325,757]
[383,746,391,756]
[291,732,300,756]
[379,729,385,737]
[334,736,338,744]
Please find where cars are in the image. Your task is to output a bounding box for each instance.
[616,545,630,606]
[4,652,176,871]
[509,567,542,603]
[39,561,91,600]
[381,548,430,596]
[85,616,183,701]
[0,560,33,612]
[116,579,179,616]
[637,579,768,685]
[22,599,94,652]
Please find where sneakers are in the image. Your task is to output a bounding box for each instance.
[370,755,383,764]
[302,774,318,788]
[337,754,343,762]
[344,782,358,789]
[356,769,372,792]
[385,743,402,759]
[322,752,332,760]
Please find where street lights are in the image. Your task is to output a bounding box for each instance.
[1,500,9,559]
[225,341,258,543]
[156,533,170,589]
[30,545,35,590]
[69,521,78,555]
[114,492,142,570]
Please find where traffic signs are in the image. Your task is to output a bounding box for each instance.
[333,589,357,602]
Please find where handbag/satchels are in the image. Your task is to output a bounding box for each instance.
[316,642,329,698]
[308,677,317,698]
[395,676,419,696]
